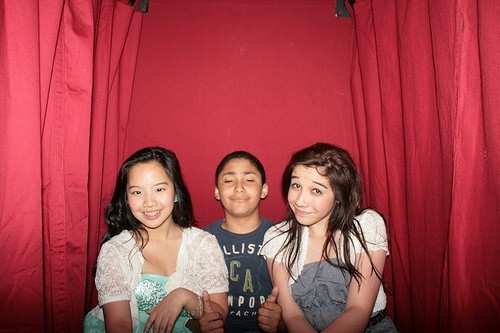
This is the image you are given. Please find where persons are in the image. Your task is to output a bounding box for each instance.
[261,141,398,333]
[81,146,230,333]
[196,150,283,333]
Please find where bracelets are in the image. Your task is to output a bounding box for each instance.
[190,295,204,318]
